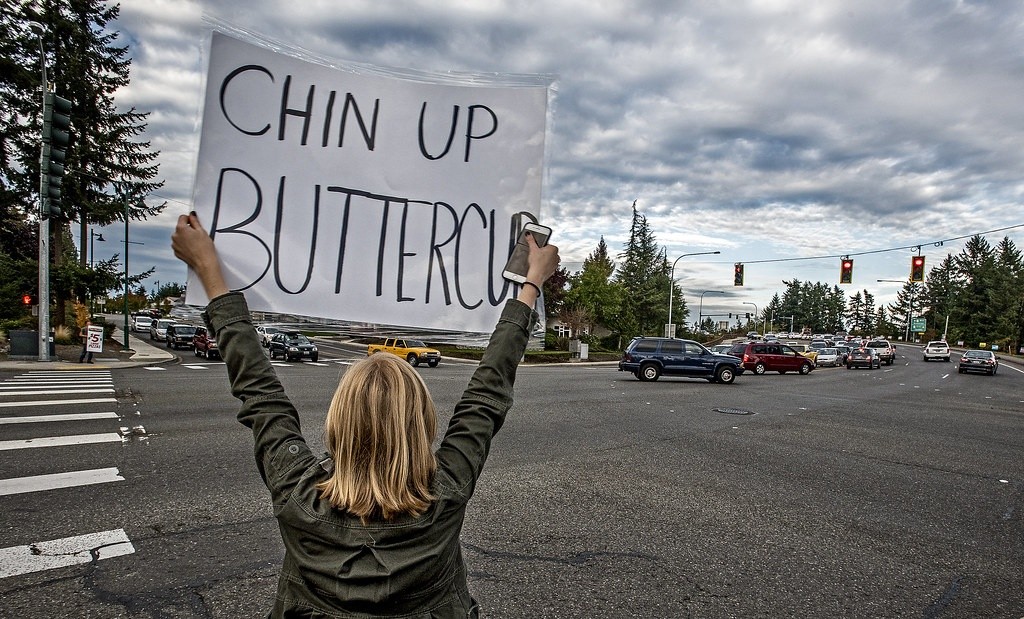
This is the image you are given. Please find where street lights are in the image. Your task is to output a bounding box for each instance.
[154,280,159,296]
[124,186,148,347]
[876,279,911,342]
[90,227,106,323]
[742,302,757,330]
[699,290,725,333]
[29,20,53,363]
[669,252,721,336]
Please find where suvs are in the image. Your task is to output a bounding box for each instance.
[742,340,816,376]
[269,331,319,362]
[193,324,224,359]
[150,317,178,342]
[864,339,895,366]
[618,332,745,385]
[166,322,198,350]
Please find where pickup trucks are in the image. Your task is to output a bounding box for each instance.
[367,336,441,368]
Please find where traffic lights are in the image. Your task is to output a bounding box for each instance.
[734,264,744,286]
[910,255,926,283]
[39,91,72,219]
[839,258,853,284]
[23,294,39,306]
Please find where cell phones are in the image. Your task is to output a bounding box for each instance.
[500,222,551,285]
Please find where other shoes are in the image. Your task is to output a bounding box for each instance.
[79,360,83,363]
[87,361,94,364]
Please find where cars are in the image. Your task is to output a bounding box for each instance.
[923,340,951,362]
[958,349,1000,375]
[131,315,153,333]
[129,303,206,327]
[255,325,287,348]
[815,347,845,368]
[708,330,885,368]
[846,346,881,370]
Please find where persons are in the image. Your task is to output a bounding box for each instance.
[170,211,560,619]
[79,320,94,364]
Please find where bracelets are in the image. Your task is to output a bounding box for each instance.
[521,282,541,297]
